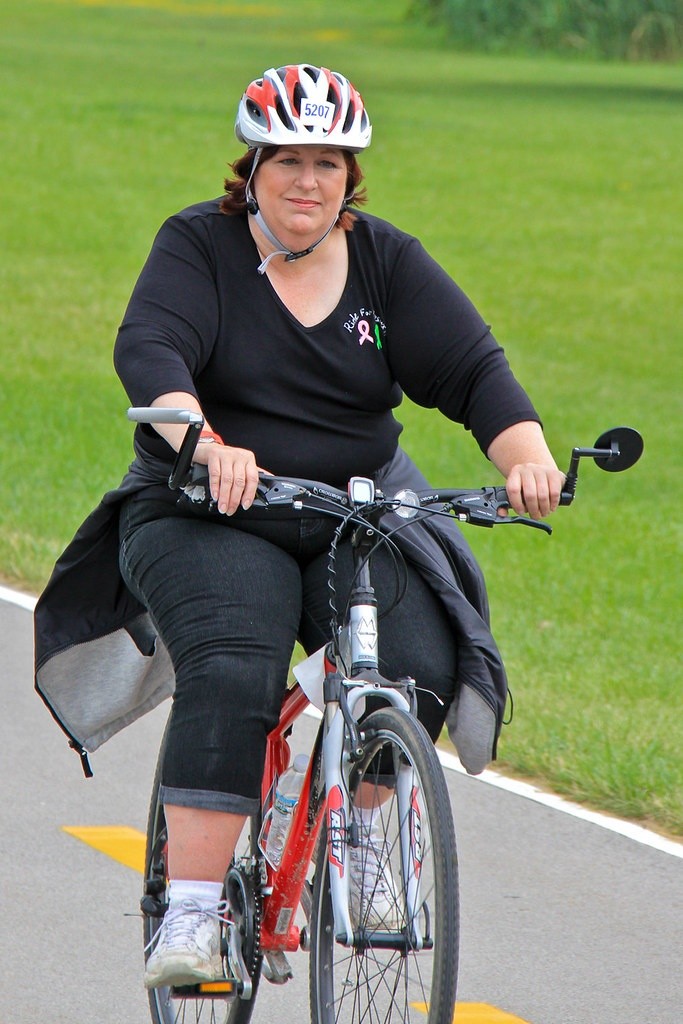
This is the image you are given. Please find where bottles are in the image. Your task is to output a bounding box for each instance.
[261,754,313,866]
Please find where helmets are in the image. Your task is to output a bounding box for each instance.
[233,62,372,153]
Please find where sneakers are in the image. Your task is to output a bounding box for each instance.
[144,909,223,990]
[345,823,407,933]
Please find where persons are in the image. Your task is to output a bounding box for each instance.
[111,59,566,990]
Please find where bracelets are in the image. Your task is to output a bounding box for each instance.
[198,430,225,446]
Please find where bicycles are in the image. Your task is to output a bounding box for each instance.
[127,403,642,1024]
